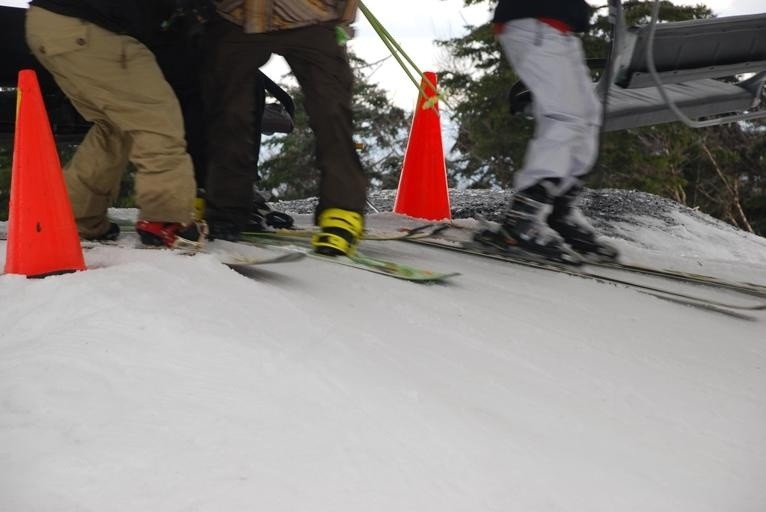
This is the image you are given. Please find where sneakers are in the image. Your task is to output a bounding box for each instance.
[252,189,294,227]
[83,223,120,240]
[317,220,352,255]
[137,223,198,246]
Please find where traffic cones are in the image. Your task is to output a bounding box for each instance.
[0,64,97,279]
[389,71,457,222]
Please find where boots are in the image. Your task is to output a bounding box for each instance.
[496,176,587,269]
[550,176,618,263]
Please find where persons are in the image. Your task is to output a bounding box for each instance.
[491,0,621,264]
[156,4,293,228]
[26,0,217,248]
[206,0,365,253]
